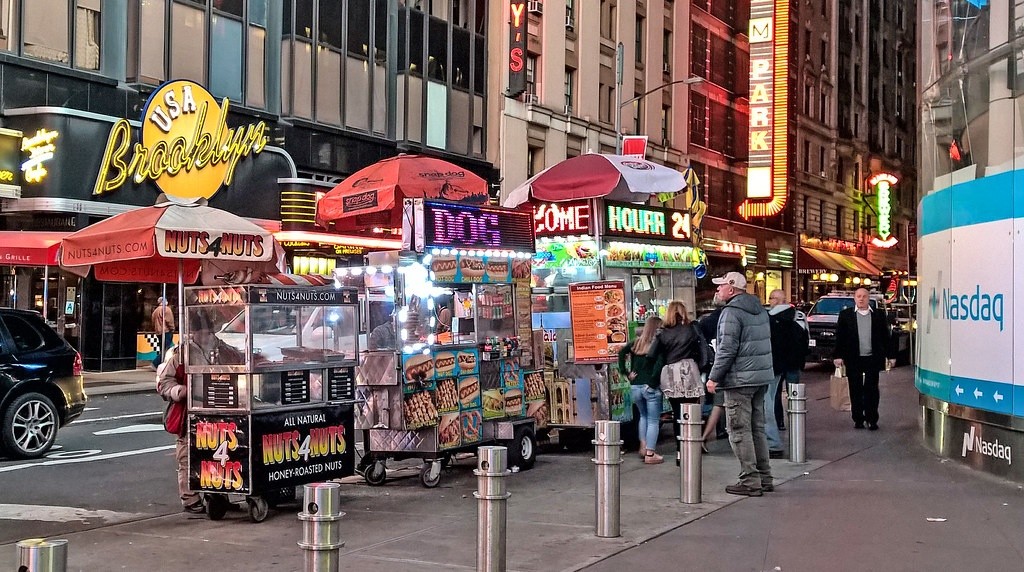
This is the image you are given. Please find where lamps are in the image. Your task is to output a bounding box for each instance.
[830,274,839,283]
[812,273,820,281]
[902,278,917,286]
[863,278,872,286]
[819,273,830,281]
[853,277,860,285]
[845,277,851,284]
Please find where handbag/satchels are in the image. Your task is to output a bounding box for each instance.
[829,366,852,412]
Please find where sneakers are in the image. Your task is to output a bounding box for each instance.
[761,481,774,492]
[725,481,763,496]
[185,501,206,513]
[227,501,239,511]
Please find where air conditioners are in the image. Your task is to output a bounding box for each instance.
[662,139,670,147]
[564,105,572,114]
[663,63,671,75]
[528,1,543,16]
[566,16,574,28]
[525,94,538,105]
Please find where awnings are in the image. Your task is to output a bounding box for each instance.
[799,247,883,276]
[0,232,70,265]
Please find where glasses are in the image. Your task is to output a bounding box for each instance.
[769,297,779,302]
[197,331,216,337]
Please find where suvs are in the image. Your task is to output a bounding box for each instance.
[0,306,88,460]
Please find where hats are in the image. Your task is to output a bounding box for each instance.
[190,313,214,331]
[711,272,746,290]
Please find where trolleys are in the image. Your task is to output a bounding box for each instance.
[357,253,698,489]
[180,283,361,525]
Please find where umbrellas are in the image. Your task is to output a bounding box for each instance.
[679,167,707,278]
[502,150,686,273]
[59,202,287,368]
[314,151,489,230]
[214,268,335,286]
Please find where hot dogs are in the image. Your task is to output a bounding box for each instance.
[405,354,432,380]
[430,256,457,275]
[459,257,485,277]
[457,351,476,369]
[460,376,479,404]
[435,352,455,372]
[487,258,509,277]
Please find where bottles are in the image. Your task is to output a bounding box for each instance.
[479,334,524,361]
[633,297,673,321]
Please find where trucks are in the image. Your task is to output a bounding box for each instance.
[805,290,910,364]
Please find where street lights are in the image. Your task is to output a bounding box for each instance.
[612,42,705,157]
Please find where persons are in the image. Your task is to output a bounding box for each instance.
[832,288,898,431]
[149,297,175,371]
[369,292,453,350]
[157,314,265,514]
[618,290,808,467]
[705,272,774,495]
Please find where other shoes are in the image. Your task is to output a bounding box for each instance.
[645,453,664,464]
[854,420,867,429]
[775,421,786,430]
[701,441,709,454]
[868,420,879,431]
[676,458,681,466]
[640,443,647,456]
[768,449,783,460]
[150,364,157,371]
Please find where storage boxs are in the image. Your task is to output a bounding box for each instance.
[281,346,345,363]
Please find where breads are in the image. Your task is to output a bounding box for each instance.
[459,410,481,439]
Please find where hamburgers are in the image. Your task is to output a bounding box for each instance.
[439,412,461,448]
[505,389,522,412]
[527,400,547,426]
[482,390,503,413]
